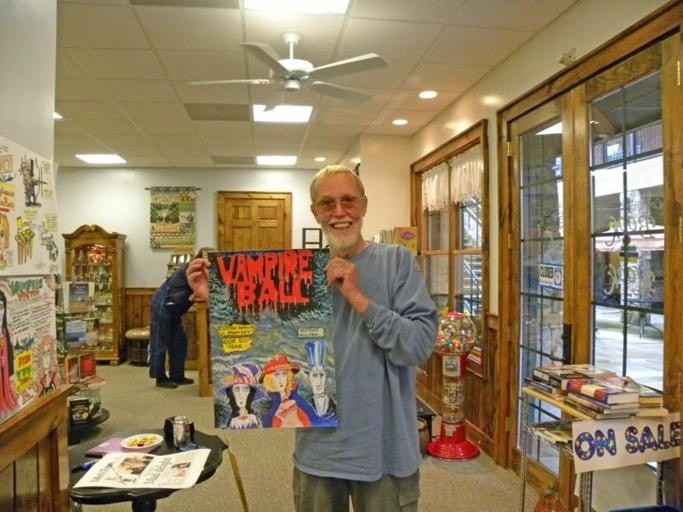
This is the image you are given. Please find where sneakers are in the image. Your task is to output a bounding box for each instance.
[156,375,194,389]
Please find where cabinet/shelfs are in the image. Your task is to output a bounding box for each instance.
[62,224,126,366]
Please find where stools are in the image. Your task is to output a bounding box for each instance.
[125,328,150,367]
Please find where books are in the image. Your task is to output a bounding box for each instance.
[525,361,663,462]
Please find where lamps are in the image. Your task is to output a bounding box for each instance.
[187,31,388,104]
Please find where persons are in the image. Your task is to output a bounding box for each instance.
[186,162,436,512]
[149,256,197,388]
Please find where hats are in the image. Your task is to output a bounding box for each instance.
[219,362,265,402]
[259,353,301,384]
[303,339,331,376]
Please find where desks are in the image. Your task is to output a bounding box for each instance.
[518,386,665,512]
[69,428,248,512]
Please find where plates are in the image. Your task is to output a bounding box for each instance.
[120,432,163,452]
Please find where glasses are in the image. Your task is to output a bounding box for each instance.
[313,195,364,210]
